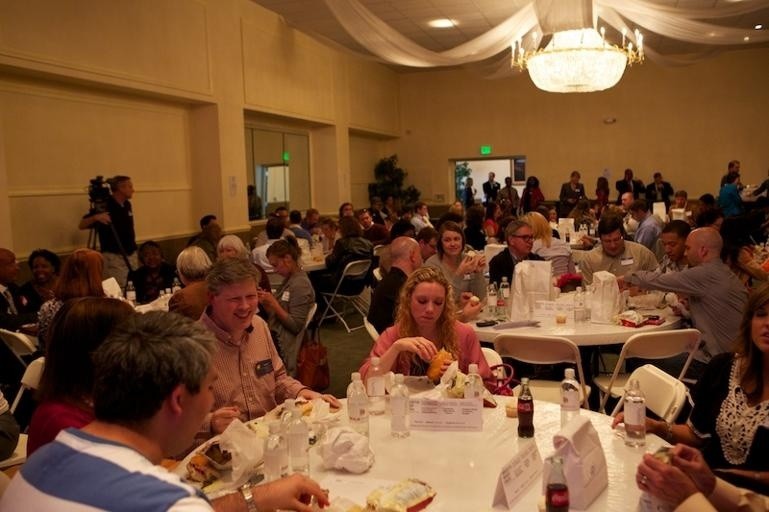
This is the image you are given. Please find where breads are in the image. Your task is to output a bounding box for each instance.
[185,454,215,483]
[427,351,453,381]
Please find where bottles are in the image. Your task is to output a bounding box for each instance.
[265,420,286,480]
[347,381,370,436]
[564,222,595,243]
[285,410,311,479]
[559,368,581,426]
[486,277,592,324]
[389,373,410,439]
[517,378,535,439]
[346,373,366,396]
[545,456,571,511]
[463,364,485,398]
[158,277,183,298]
[277,398,296,478]
[365,357,386,416]
[126,280,136,308]
[624,378,646,449]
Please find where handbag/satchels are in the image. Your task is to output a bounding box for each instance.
[295,323,330,393]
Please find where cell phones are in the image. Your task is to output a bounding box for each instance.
[642,315,659,320]
[477,319,495,327]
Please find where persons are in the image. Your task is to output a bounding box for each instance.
[1,160,769,511]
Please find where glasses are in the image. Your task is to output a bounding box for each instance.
[512,234,534,243]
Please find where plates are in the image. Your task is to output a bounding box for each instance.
[310,474,395,510]
[263,399,348,423]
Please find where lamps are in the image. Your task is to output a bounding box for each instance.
[509,24,646,94]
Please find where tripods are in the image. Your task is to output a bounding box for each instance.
[87,223,134,276]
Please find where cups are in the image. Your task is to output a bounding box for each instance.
[506,397,519,420]
[310,242,323,262]
